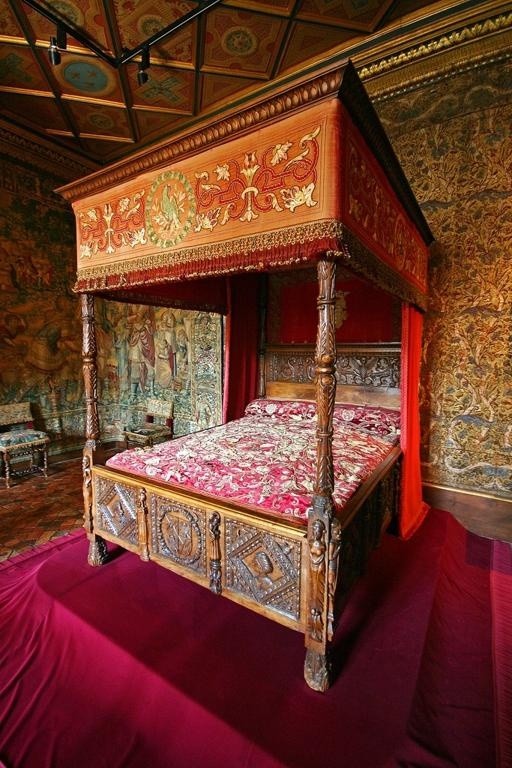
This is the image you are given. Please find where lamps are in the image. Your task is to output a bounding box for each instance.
[24,1,231,84]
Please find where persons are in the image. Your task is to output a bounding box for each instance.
[2,314,26,348]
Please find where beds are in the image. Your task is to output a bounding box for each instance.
[50,55,438,691]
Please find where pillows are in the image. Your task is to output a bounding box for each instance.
[243,399,317,421]
[333,403,401,436]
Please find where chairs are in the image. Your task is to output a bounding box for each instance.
[1,401,50,488]
[124,400,173,448]
